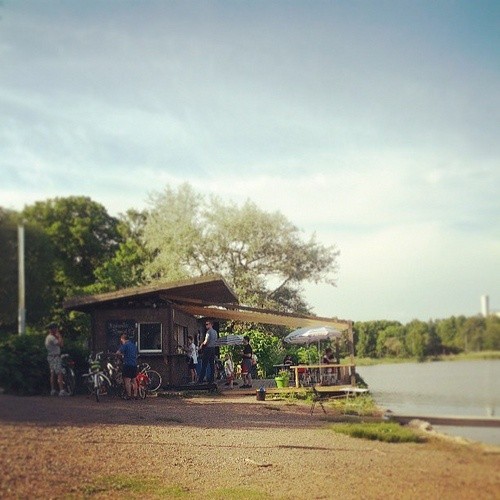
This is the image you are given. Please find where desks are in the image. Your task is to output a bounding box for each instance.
[293,365,355,388]
[273,365,291,382]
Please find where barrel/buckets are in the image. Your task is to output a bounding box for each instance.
[256,390,266,401]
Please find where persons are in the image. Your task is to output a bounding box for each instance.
[240,336,253,388]
[284,354,293,365]
[185,336,198,384]
[43,321,68,397]
[116,335,139,399]
[323,348,338,364]
[197,320,217,384]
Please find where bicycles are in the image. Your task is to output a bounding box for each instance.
[57,350,77,398]
[82,347,125,403]
[130,360,163,400]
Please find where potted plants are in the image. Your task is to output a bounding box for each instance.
[274,370,291,387]
[256,385,267,400]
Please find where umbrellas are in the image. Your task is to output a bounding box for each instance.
[284,326,344,344]
[215,334,246,345]
